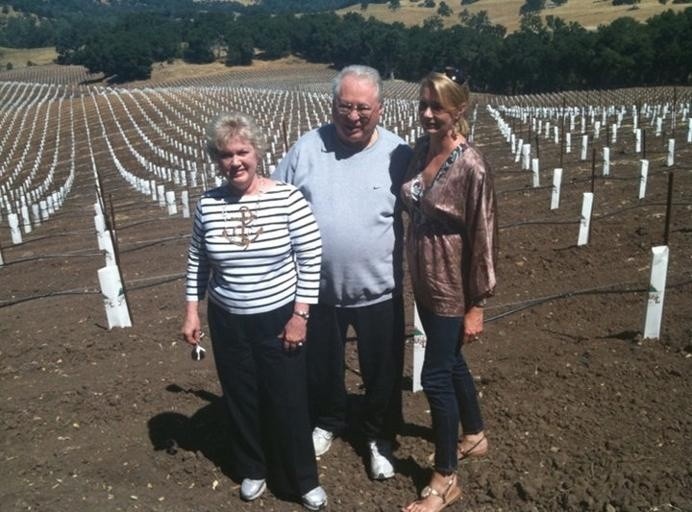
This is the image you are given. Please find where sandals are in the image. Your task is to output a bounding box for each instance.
[426,435,489,469]
[399,471,463,512]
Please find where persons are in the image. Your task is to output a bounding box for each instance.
[392,65,503,510]
[178,110,329,512]
[261,65,424,480]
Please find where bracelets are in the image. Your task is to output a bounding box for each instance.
[294,312,310,319]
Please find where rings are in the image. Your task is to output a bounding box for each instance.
[298,342,303,346]
[475,337,478,339]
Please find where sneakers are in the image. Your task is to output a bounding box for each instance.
[298,486,330,512]
[365,439,397,481]
[311,425,334,459]
[239,474,269,501]
